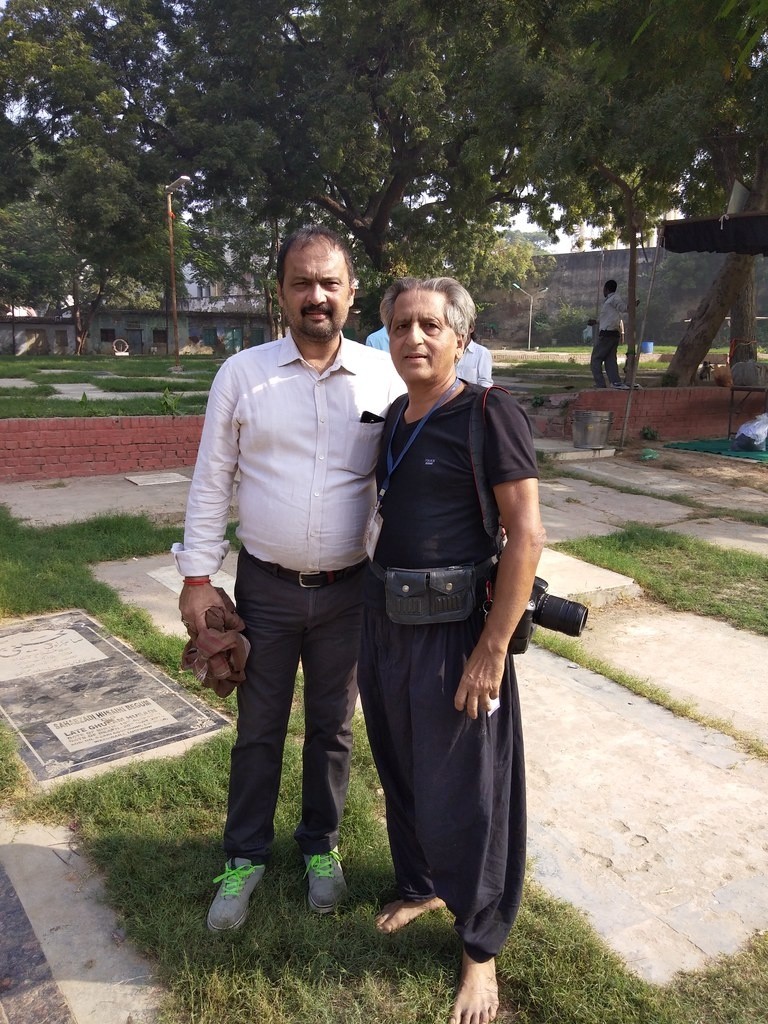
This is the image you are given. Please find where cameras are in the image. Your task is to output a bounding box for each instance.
[507,575,589,655]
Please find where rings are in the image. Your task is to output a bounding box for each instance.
[183,620,189,627]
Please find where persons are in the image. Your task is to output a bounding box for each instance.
[366,325,390,353]
[588,280,640,388]
[455,325,494,388]
[171,224,409,933]
[356,277,544,1024]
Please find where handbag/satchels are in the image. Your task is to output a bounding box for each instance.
[370,556,499,624]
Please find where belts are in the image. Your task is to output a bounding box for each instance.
[253,556,364,589]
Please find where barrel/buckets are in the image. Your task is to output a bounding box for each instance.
[640,341,654,354]
[570,409,614,450]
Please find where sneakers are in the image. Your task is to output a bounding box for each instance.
[206,857,266,931]
[302,845,346,913]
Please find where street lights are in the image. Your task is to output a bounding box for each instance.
[164,176,191,370]
[512,284,548,351]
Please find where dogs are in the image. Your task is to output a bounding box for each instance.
[713,362,733,386]
[699,360,710,380]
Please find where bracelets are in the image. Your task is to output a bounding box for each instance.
[183,580,211,584]
